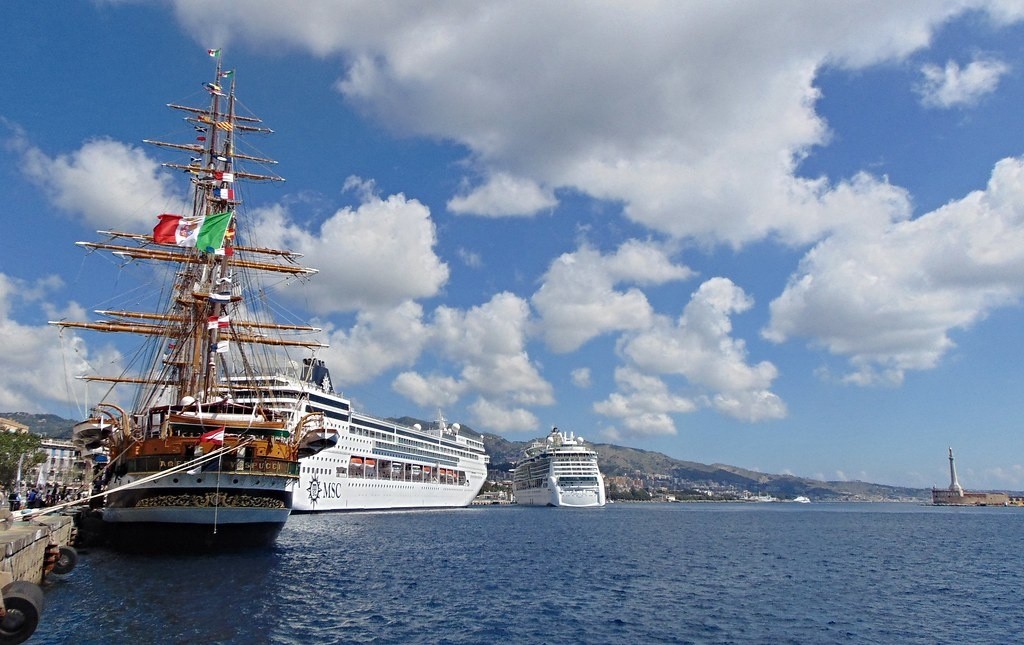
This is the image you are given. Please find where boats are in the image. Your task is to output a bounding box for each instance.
[392,463,401,473]
[440,470,445,476]
[366,459,376,468]
[349,457,362,467]
[423,467,429,474]
[448,471,453,478]
[413,465,421,476]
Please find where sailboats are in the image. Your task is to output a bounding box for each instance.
[47,46,339,551]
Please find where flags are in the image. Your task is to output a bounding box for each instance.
[208,48,221,59]
[214,188,234,200]
[189,115,232,182]
[218,70,235,80]
[154,211,234,257]
[206,292,230,354]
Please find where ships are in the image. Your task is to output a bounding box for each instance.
[216,354,492,514]
[510,428,609,510]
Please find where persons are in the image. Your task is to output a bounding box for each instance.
[0,483,86,512]
